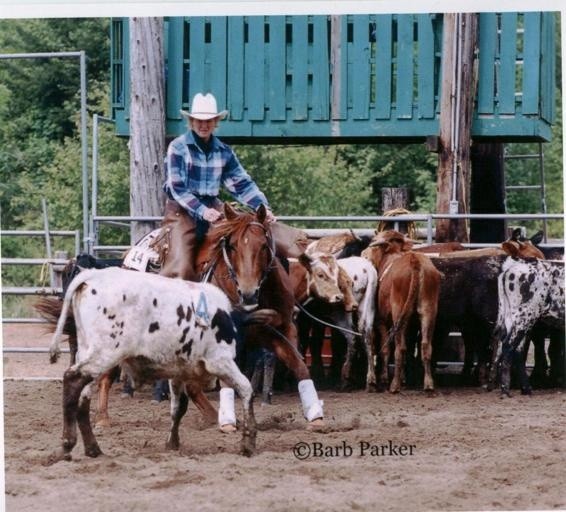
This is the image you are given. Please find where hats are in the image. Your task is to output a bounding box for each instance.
[180,92,228,121]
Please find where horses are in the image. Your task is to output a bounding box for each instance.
[30,201,329,434]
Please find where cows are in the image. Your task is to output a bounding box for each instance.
[223,206,566,400]
[53,253,169,406]
[49,266,279,462]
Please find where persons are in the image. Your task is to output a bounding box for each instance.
[158,92,277,282]
